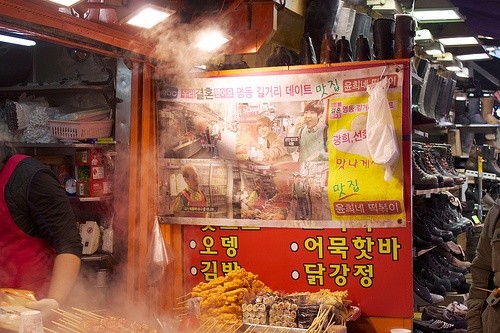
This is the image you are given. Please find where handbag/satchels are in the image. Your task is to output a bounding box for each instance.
[266,45,299,66]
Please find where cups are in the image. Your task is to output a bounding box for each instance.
[390,329,412,333]
[19,310,44,333]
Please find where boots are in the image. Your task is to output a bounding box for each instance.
[412,0,500,126]
[301,15,415,65]
[411,138,500,188]
[412,191,480,333]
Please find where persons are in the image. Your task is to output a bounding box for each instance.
[294,100,328,187]
[173,166,208,218]
[0,137,84,305]
[253,116,294,166]
[467,193,500,333]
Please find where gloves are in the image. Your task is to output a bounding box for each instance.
[24,298,60,326]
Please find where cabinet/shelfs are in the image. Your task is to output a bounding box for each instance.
[0,84,116,263]
[410,71,500,333]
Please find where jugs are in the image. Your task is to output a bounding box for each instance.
[372,18,396,60]
[394,16,416,59]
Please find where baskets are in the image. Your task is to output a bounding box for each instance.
[48,118,114,140]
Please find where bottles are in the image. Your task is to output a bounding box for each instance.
[355,35,371,61]
[337,36,350,62]
